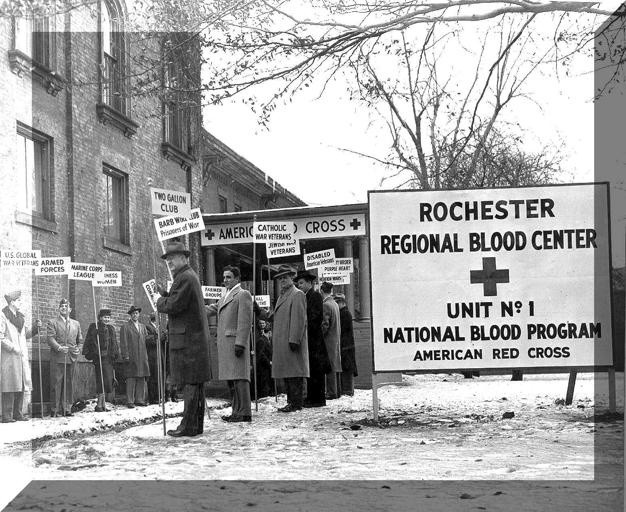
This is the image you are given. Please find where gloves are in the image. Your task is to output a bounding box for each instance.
[235,345,244,357]
[59,345,69,356]
[155,284,165,295]
[123,356,131,362]
[91,327,99,337]
[160,334,167,342]
[35,319,42,329]
[286,343,299,351]
[154,333,158,340]
[12,346,22,355]
[253,300,262,313]
[149,291,162,302]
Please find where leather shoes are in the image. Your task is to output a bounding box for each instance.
[95,406,104,412]
[171,398,178,402]
[50,412,62,417]
[278,403,302,411]
[128,403,135,408]
[136,403,149,406]
[222,415,251,422]
[63,412,73,416]
[16,416,29,421]
[167,428,192,437]
[2,419,16,423]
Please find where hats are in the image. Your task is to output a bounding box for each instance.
[99,309,112,315]
[294,271,316,280]
[59,298,71,304]
[4,291,21,302]
[128,306,141,314]
[160,243,190,257]
[334,293,345,302]
[273,264,296,278]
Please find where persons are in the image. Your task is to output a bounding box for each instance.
[258,320,265,331]
[146,312,167,404]
[82,309,119,412]
[205,265,253,422]
[0,291,42,422]
[255,265,309,412]
[47,299,82,417]
[292,269,331,408]
[120,306,149,409]
[256,329,273,400]
[333,293,357,396]
[151,243,212,437]
[319,282,342,400]
